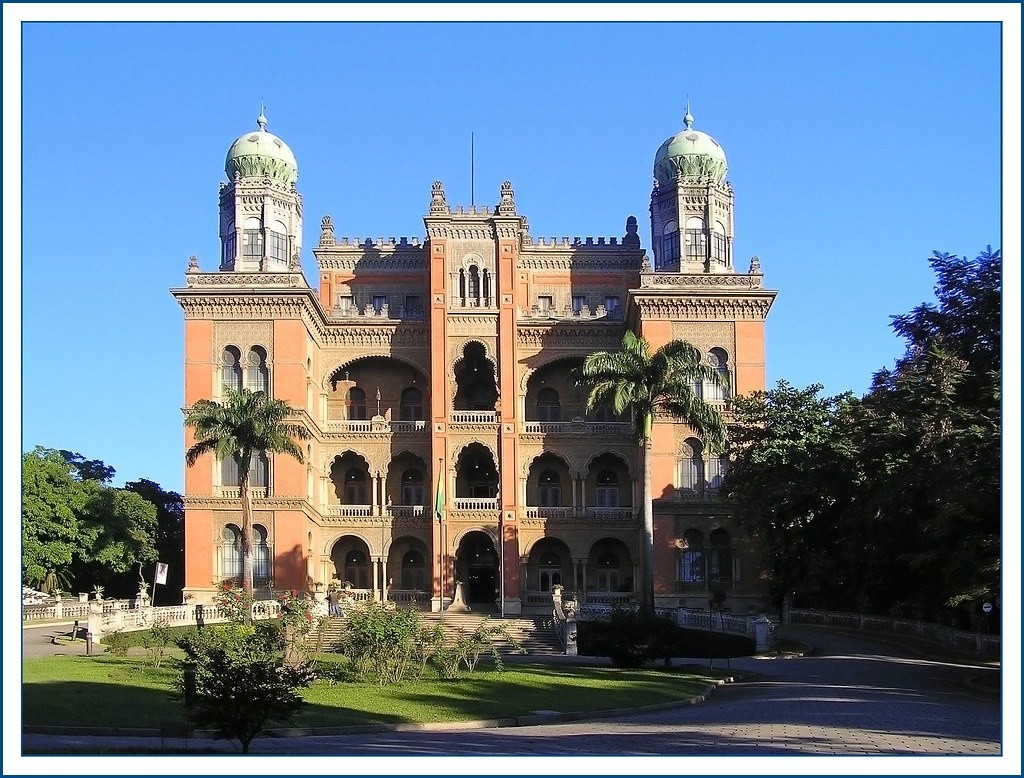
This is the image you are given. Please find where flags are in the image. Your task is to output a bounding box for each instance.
[436,462,443,524]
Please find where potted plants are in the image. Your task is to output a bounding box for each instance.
[138,581,150,593]
[562,601,577,617]
[551,584,564,595]
[90,585,105,599]
[51,588,63,601]
[709,575,733,602]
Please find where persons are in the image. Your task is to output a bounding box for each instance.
[329,589,340,617]
